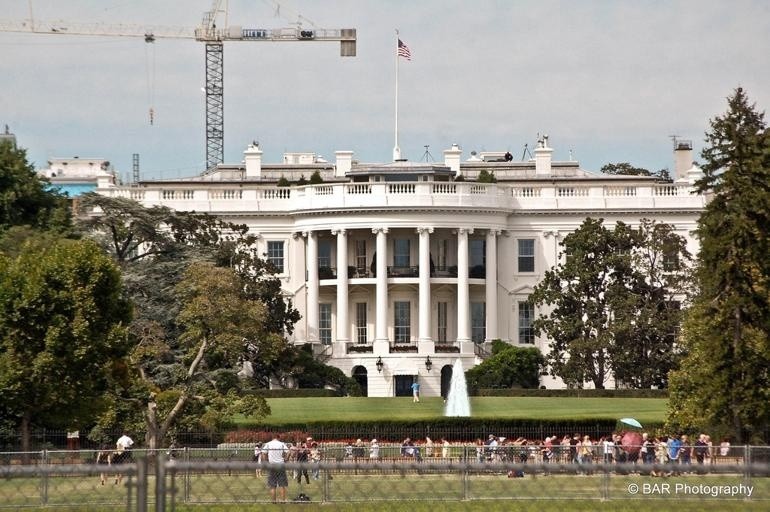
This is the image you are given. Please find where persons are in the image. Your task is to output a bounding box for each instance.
[343,439,379,458]
[255,434,320,504]
[400,435,450,457]
[96,431,135,485]
[475,431,730,477]
[411,380,419,402]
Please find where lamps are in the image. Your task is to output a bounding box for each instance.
[425,356,433,372]
[376,356,384,373]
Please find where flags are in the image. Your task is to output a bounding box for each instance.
[398,37,411,62]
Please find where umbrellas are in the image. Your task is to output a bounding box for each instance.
[619,417,642,428]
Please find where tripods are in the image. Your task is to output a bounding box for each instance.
[522,147,533,162]
[418,147,436,163]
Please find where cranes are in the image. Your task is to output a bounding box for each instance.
[0,1,357,168]
